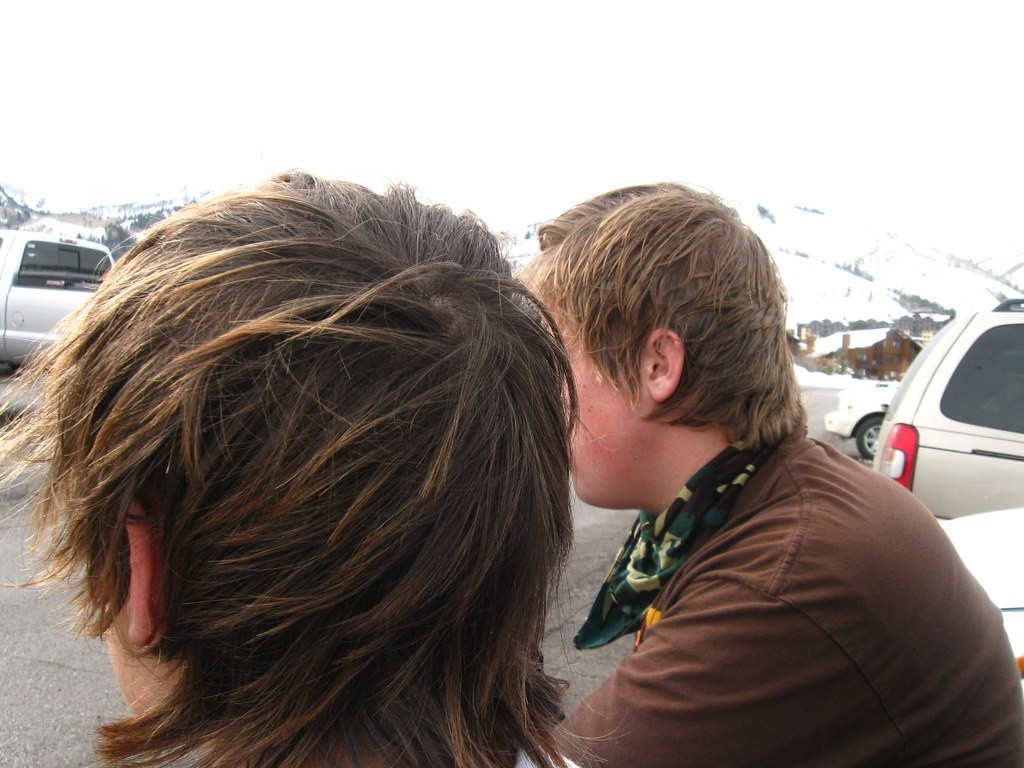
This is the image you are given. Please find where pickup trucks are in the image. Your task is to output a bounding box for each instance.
[0,227,127,368]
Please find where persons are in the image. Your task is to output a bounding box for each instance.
[0,170,583,768]
[521,181,1023,768]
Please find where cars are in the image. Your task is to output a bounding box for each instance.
[868,295,1024,527]
[936,507,1024,687]
[820,383,896,459]
